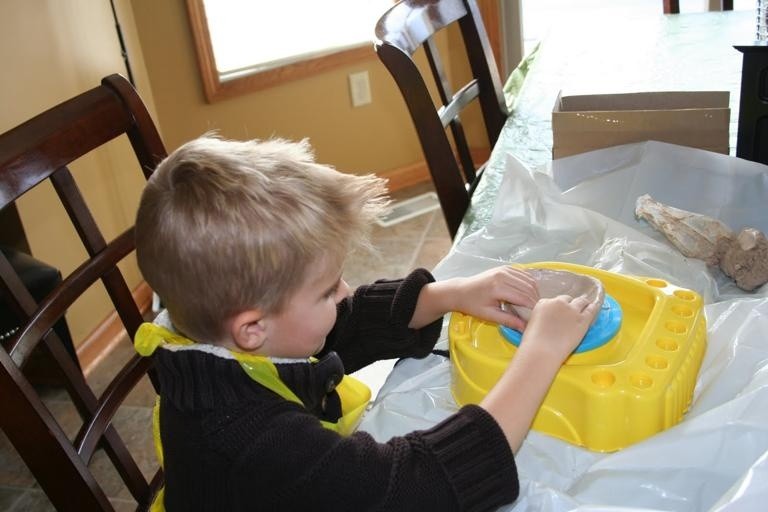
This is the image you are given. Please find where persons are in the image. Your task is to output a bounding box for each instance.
[129,128,599,510]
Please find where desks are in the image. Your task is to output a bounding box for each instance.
[353,30,766,511]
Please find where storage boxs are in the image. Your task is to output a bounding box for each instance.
[550,91,730,160]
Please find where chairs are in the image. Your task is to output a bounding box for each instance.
[0,74,171,511]
[371,1,511,241]
